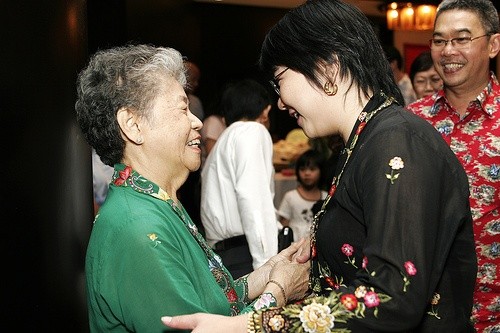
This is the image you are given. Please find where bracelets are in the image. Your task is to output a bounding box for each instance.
[266,280,286,306]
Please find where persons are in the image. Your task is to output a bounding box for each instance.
[161,0,478,333]
[76,44,310,333]
[381,44,444,108]
[178,74,328,280]
[405,0,500,333]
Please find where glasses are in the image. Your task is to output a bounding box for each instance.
[429,32,495,50]
[270,67,289,95]
